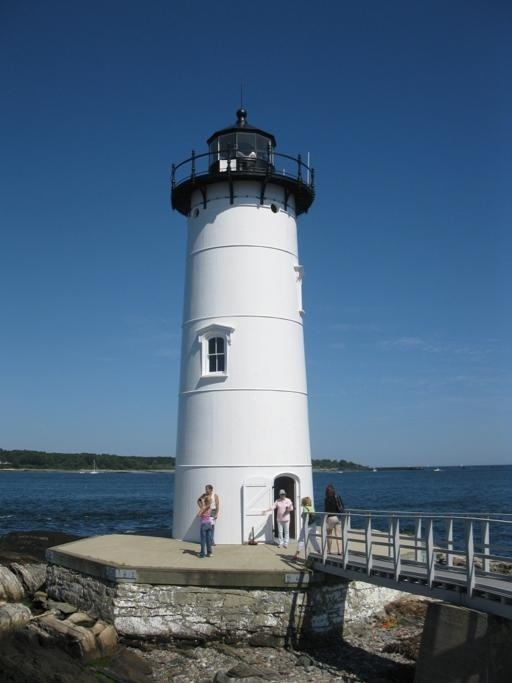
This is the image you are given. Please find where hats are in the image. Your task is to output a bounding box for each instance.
[278,489,287,495]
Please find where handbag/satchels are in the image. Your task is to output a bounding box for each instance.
[338,511,346,520]
[309,513,317,524]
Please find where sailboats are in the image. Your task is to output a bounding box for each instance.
[80,459,104,474]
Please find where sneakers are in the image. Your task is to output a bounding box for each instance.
[206,553,215,557]
[196,552,205,558]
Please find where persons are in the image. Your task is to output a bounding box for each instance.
[262,489,294,548]
[290,496,321,561]
[324,485,345,555]
[197,485,219,558]
